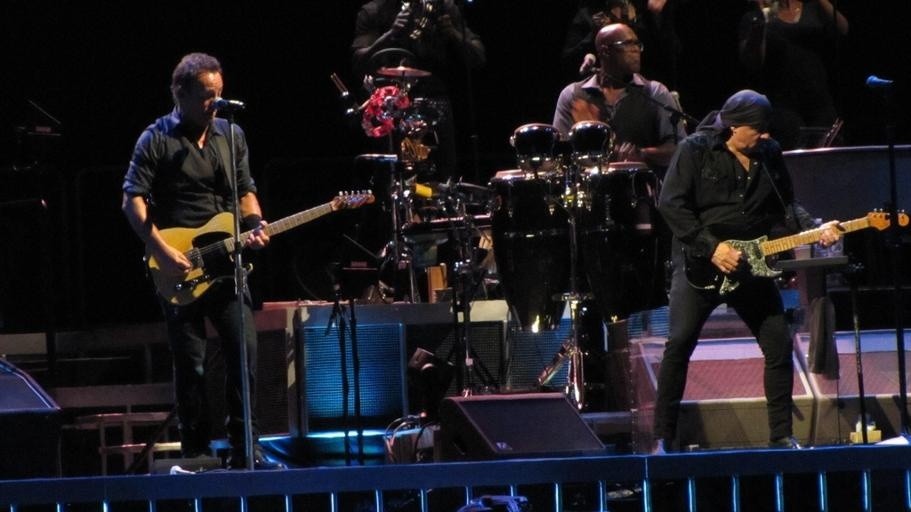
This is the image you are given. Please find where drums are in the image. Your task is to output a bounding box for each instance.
[513,124,559,171]
[578,162,659,242]
[567,121,615,167]
[489,170,571,241]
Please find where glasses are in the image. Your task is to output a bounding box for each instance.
[610,39,643,52]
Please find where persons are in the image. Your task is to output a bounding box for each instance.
[548,23,693,174]
[556,1,685,80]
[650,89,845,451]
[121,46,291,473]
[731,0,858,152]
[349,1,490,81]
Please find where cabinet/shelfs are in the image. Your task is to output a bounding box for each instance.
[72,411,234,476]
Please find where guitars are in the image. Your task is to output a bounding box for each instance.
[146,191,375,305]
[715,207,909,293]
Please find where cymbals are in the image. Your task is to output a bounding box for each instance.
[378,67,431,76]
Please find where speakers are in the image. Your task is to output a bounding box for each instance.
[796,326,910,447]
[633,334,818,450]
[0,357,62,478]
[437,390,604,460]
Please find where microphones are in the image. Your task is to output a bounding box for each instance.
[580,55,596,74]
[212,97,246,111]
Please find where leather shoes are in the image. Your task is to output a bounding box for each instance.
[228,444,289,470]
[650,439,674,453]
[768,435,806,450]
